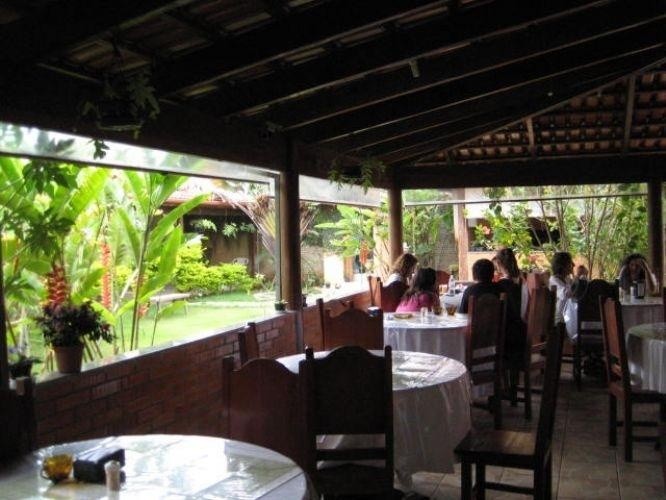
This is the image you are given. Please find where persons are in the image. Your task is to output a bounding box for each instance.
[616,253,659,294]
[546,252,607,378]
[383,253,418,287]
[458,258,503,314]
[395,268,441,313]
[495,248,528,310]
[490,257,501,278]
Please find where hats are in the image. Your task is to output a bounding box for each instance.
[550,253,574,267]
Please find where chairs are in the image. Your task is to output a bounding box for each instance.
[595,294,666,464]
[434,271,450,290]
[237,322,260,367]
[510,285,558,421]
[453,322,567,500]
[315,298,355,346]
[220,356,310,465]
[467,295,508,430]
[574,279,620,392]
[323,308,384,352]
[534,271,551,288]
[301,347,398,499]
[366,276,386,313]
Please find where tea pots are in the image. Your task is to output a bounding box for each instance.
[40,453,73,485]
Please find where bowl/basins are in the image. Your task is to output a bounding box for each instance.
[433,307,443,315]
[445,306,458,317]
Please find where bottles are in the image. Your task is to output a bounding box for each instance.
[449,274,455,295]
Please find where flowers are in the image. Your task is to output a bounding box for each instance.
[31,299,116,346]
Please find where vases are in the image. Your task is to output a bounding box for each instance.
[53,344,84,375]
